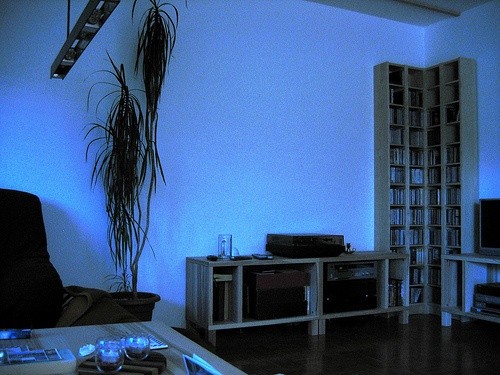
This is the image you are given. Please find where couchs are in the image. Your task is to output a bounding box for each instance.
[0,188,64,328]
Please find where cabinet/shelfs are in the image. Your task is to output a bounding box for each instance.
[373,57,500,327]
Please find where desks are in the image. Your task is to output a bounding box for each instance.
[184,255,319,346]
[319,251,410,337]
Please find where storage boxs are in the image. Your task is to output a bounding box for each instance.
[243,268,307,320]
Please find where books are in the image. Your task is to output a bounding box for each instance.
[390,86,463,308]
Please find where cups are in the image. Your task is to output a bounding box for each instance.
[124,332,150,360]
[95,336,124,373]
[218,233,232,260]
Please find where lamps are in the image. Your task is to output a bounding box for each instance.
[50,0,121,80]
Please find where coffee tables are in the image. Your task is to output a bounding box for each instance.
[0,321,251,375]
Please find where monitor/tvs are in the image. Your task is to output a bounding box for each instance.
[478,198,500,259]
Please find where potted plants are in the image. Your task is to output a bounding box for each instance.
[81,0,188,324]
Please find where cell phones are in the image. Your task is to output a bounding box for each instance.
[252,254,273,260]
